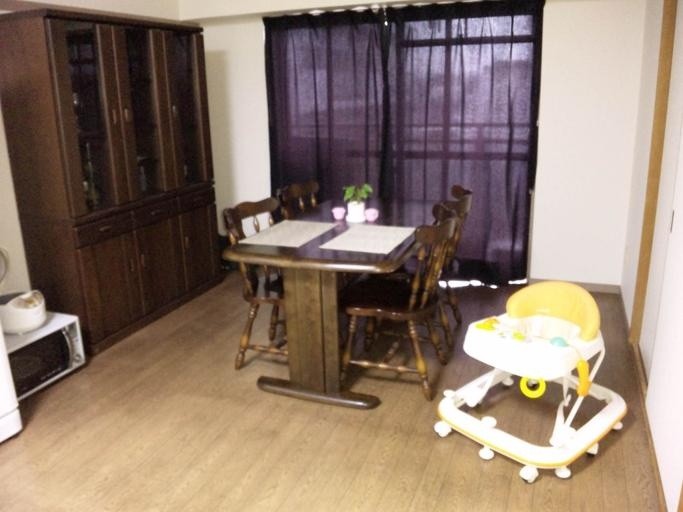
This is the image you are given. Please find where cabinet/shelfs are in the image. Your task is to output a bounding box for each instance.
[0,7,225,357]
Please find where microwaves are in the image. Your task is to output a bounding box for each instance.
[5,308,87,404]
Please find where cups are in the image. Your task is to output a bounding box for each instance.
[366,208,379,222]
[332,207,345,220]
[333,222,346,234]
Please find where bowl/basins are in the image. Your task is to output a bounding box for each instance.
[1,287,48,335]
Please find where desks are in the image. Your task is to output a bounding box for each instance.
[223,196,450,409]
